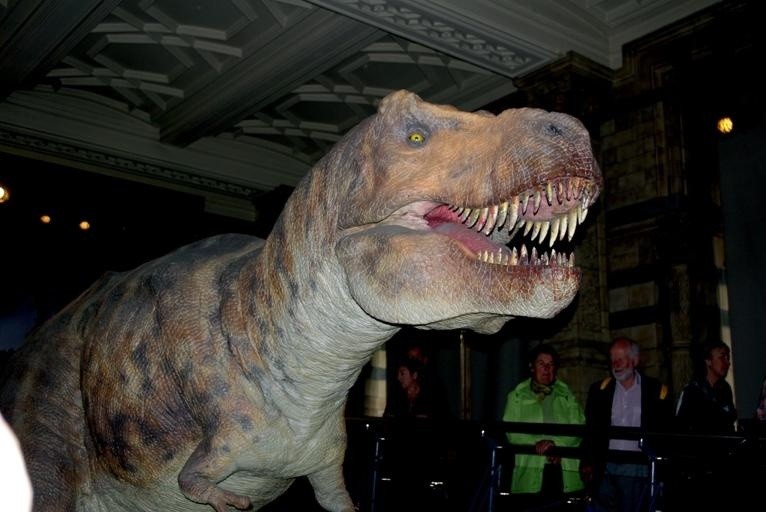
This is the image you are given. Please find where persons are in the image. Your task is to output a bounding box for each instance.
[579,339,675,512]
[674,337,739,512]
[500,345,589,512]
[346,344,451,512]
